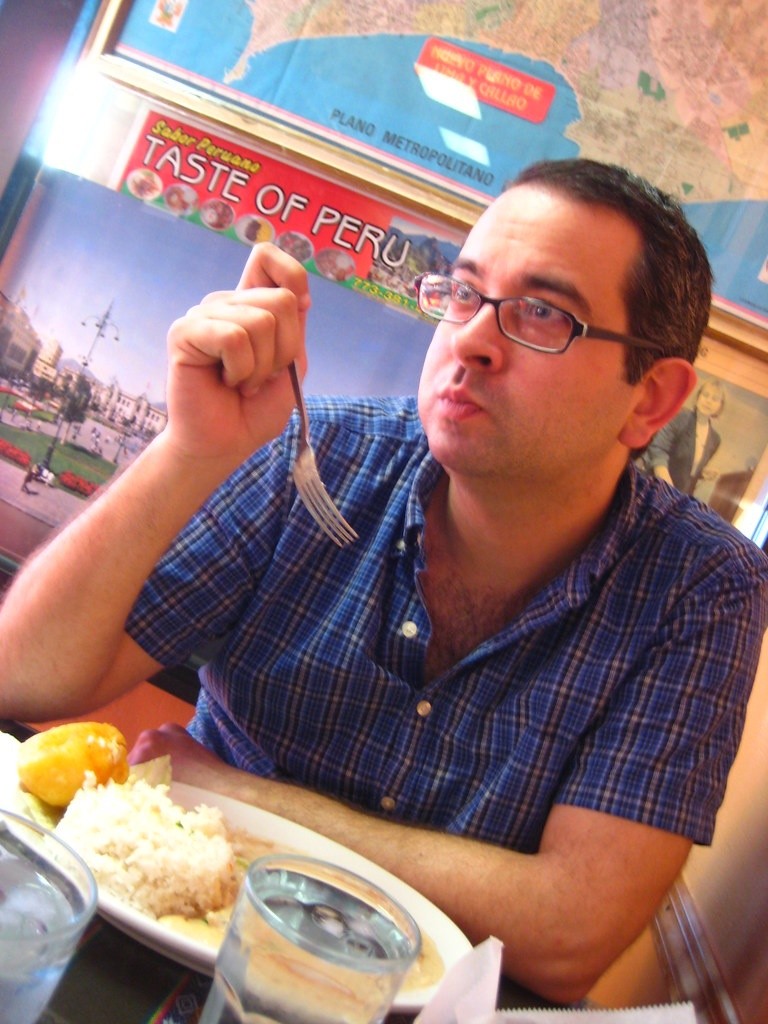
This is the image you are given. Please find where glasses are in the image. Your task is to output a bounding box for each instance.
[413,271,665,358]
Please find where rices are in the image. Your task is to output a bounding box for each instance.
[37,769,249,925]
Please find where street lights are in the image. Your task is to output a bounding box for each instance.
[25,297,122,490]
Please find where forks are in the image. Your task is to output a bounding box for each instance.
[288,361,356,548]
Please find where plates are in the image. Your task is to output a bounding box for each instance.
[26,779,473,1013]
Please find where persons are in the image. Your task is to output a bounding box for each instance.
[0,158,768,1004]
[640,381,727,496]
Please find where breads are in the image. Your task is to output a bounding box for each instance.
[12,721,130,807]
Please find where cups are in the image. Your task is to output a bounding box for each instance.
[200,855,420,1023]
[0,809,97,1023]
